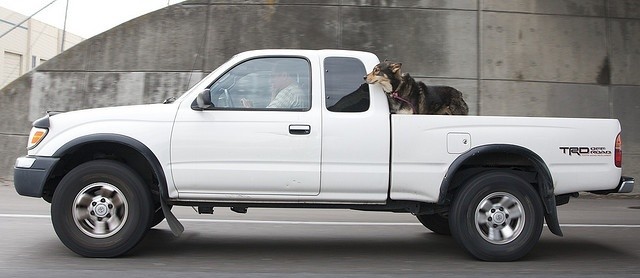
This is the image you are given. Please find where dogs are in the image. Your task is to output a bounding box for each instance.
[363,58,469,116]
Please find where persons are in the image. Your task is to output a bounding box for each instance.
[239,61,305,110]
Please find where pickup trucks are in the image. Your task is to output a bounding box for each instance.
[15,49,633,261]
